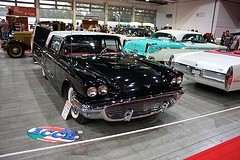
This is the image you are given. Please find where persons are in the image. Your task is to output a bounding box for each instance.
[9,18,16,31]
[19,21,25,32]
[220,29,231,46]
[0,18,7,39]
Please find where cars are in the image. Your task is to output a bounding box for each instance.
[5,20,240,97]
[32,29,186,124]
